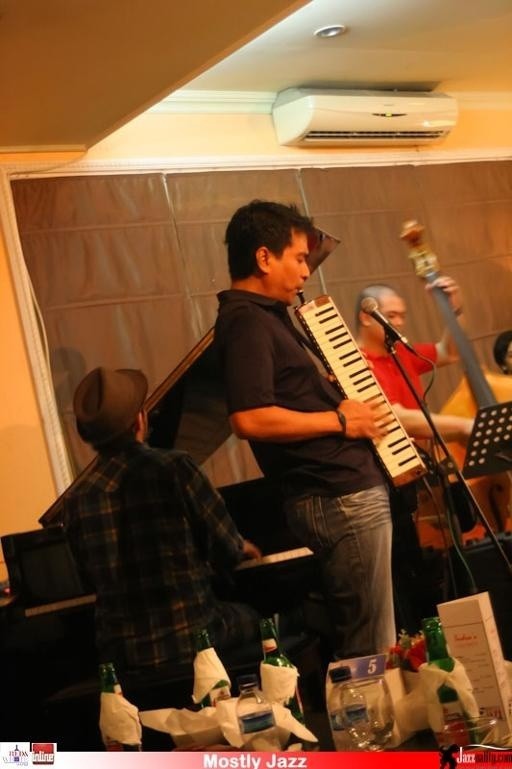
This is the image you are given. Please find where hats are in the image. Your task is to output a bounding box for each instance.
[73,366,147,446]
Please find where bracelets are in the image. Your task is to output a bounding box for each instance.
[454,305,463,318]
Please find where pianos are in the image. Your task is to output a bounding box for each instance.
[295,294,427,489]
[0,222,422,751]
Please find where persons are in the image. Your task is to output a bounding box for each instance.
[58,364,267,752]
[212,196,404,673]
[488,329,512,379]
[347,274,500,452]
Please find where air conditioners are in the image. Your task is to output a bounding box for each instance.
[272,86,460,150]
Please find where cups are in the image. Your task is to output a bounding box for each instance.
[339,676,395,752]
[442,714,499,749]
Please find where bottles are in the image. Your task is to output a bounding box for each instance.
[96,660,142,751]
[419,615,478,748]
[234,674,284,751]
[193,627,232,709]
[326,666,370,752]
[257,616,306,726]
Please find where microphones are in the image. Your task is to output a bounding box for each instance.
[360,294,420,359]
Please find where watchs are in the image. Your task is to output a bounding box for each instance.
[336,410,347,435]
